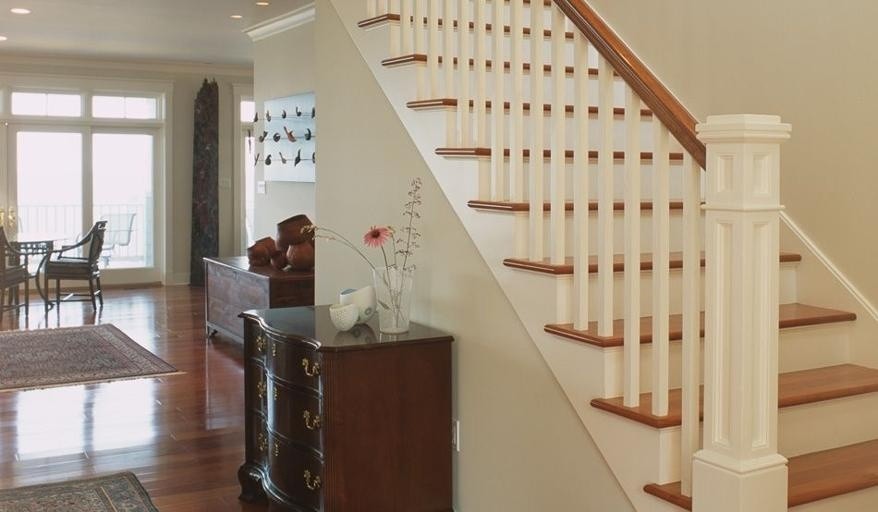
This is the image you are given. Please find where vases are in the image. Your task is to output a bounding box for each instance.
[373,265,413,335]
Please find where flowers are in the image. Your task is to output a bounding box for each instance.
[301,175,423,328]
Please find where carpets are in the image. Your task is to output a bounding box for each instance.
[0,471,160,512]
[0,323,188,391]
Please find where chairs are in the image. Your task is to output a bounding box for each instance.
[0,220,108,315]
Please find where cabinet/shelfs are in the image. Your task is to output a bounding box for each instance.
[201,255,314,348]
[236,308,453,511]
